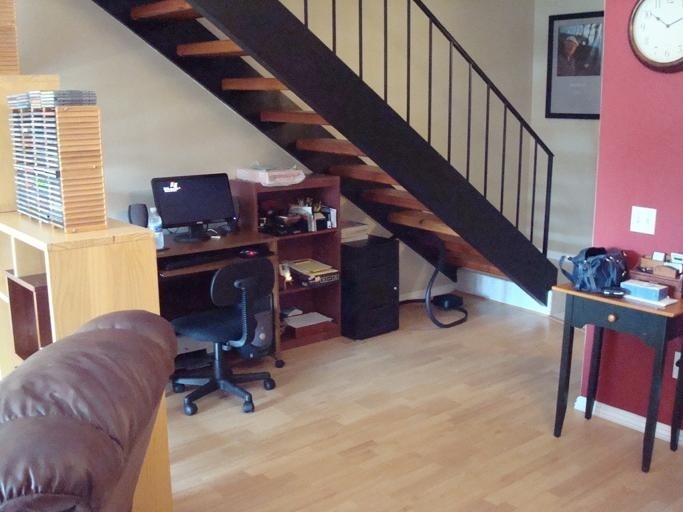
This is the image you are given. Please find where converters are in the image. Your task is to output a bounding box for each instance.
[432,294,462,309]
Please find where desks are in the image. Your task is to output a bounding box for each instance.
[551,282,683,472]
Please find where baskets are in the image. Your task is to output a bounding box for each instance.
[628,255,682,300]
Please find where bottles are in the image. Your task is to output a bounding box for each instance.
[148,207,164,250]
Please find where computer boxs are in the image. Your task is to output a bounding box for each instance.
[237,291,277,362]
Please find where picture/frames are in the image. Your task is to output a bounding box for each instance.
[545,10,604,119]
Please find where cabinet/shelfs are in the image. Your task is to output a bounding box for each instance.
[0,72,177,512]
[157,172,342,368]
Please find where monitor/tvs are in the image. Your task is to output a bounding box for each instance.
[151,173,236,244]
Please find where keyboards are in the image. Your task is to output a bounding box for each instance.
[160,249,234,271]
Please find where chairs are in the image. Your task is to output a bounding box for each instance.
[168,258,276,416]
[0,310,179,512]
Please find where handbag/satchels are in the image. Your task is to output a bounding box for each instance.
[557,247,626,296]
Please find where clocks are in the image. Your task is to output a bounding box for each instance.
[628,0,683,72]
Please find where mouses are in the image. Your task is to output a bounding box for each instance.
[239,247,257,257]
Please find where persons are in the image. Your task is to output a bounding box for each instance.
[557,35,579,75]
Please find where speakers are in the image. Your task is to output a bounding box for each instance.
[222,198,240,232]
[342,236,399,338]
